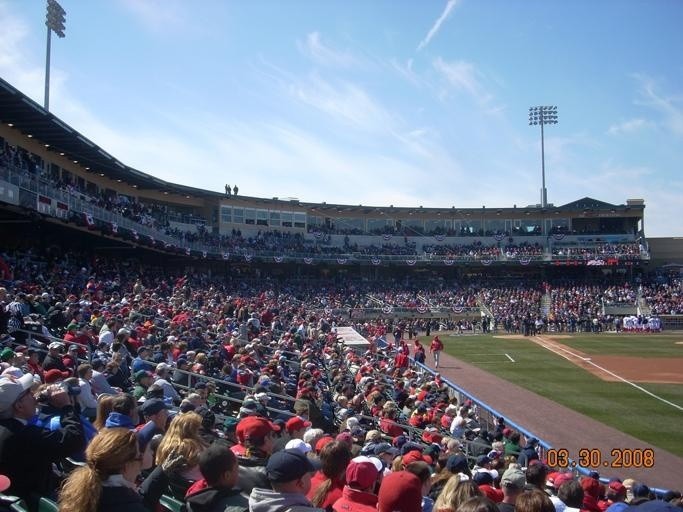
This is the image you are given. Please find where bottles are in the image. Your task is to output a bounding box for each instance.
[33,381,66,403]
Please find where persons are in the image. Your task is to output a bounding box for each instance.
[1,224,683,511]
[227,185,231,195]
[224,184,228,194]
[232,185,238,195]
[0,141,202,224]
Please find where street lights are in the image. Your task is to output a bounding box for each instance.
[528,103,559,211]
[43,0,66,111]
